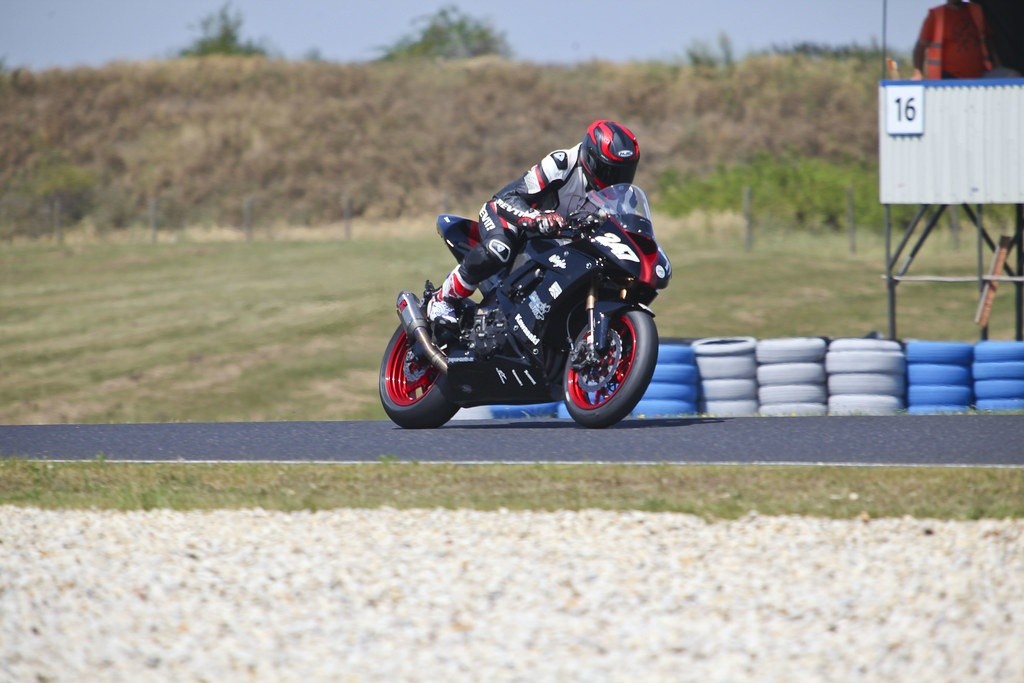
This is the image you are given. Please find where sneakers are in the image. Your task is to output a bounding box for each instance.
[427,296,457,325]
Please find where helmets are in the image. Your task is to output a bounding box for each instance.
[579,120,640,200]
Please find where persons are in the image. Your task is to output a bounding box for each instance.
[426,118,639,326]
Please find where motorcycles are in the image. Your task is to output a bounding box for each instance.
[376,181,673,426]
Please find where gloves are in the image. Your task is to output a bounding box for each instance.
[517,208,568,239]
